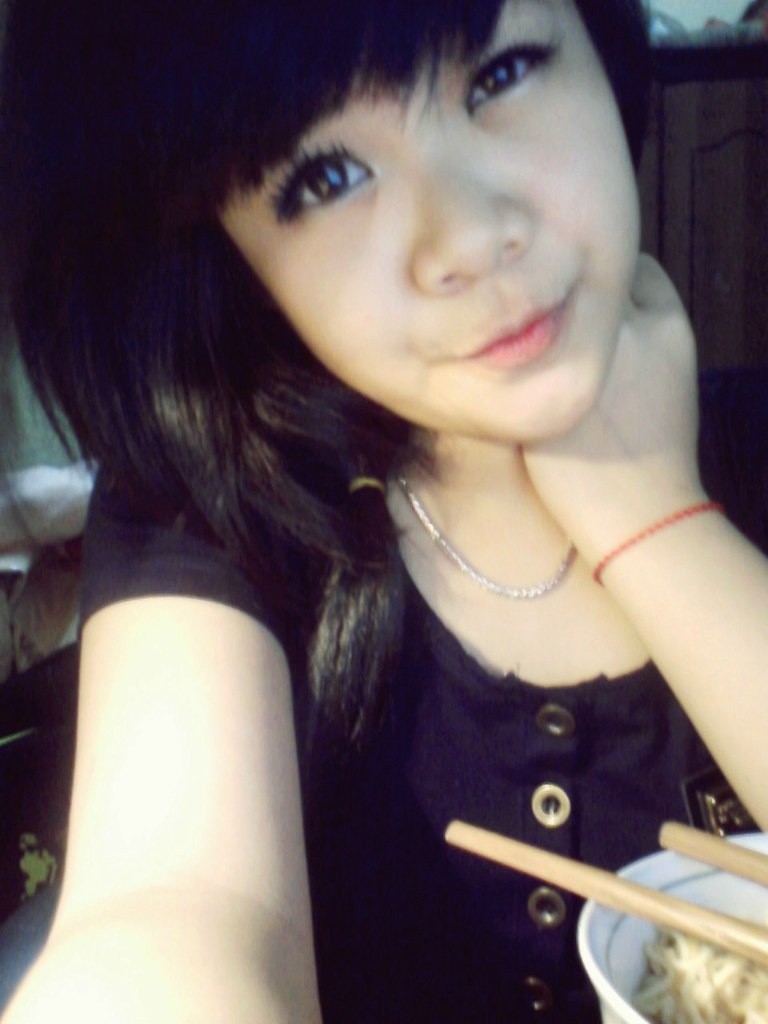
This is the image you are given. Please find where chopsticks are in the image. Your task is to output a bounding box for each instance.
[446,821,768,965]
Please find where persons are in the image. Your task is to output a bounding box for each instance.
[0,0,768,1024]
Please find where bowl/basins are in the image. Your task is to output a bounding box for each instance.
[576,832,768,1024]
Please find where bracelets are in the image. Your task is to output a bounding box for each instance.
[593,502,724,583]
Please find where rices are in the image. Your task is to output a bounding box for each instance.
[627,928,768,1024]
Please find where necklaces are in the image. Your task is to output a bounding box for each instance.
[393,467,573,598]
